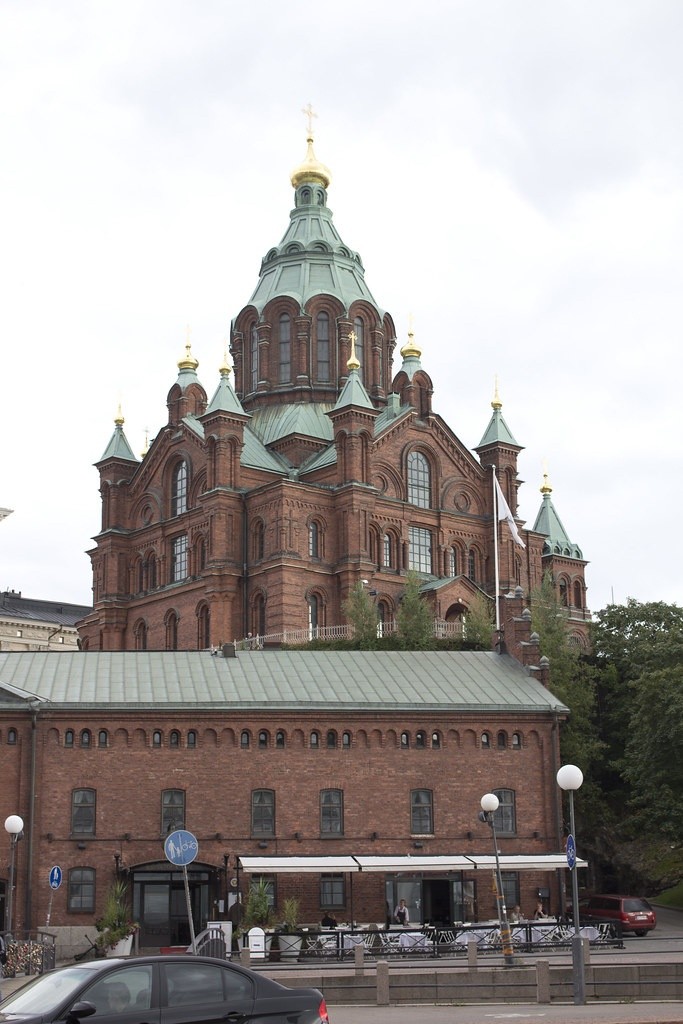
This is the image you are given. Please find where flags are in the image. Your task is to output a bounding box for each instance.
[495,475,526,549]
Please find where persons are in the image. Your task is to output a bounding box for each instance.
[509,905,525,923]
[322,911,338,930]
[533,902,548,920]
[393,899,409,926]
[107,983,137,1013]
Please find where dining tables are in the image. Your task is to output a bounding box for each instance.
[318,919,602,955]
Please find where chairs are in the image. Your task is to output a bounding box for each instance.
[301,928,319,955]
[316,916,610,960]
[80,969,243,1016]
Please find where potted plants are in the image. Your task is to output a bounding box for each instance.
[95,868,141,958]
[278,895,304,958]
[231,871,283,963]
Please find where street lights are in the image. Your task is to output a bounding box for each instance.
[4,815,25,949]
[479,793,515,966]
[556,764,587,1003]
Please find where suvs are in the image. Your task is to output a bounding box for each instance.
[564,894,657,937]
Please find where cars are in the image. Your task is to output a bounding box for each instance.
[0,954,329,1024]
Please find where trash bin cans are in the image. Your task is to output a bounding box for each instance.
[247,927,265,959]
